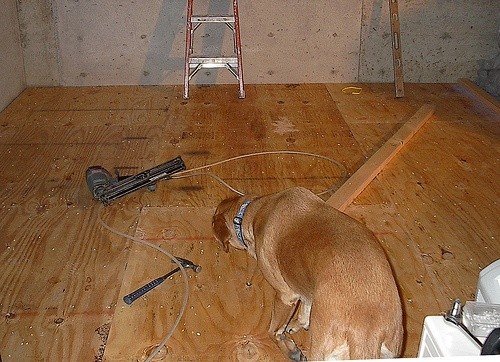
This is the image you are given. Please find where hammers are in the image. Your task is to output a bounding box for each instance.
[123,256,202,305]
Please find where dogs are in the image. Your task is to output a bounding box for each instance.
[212,186,405,362]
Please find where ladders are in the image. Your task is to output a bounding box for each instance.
[183,1,246,99]
